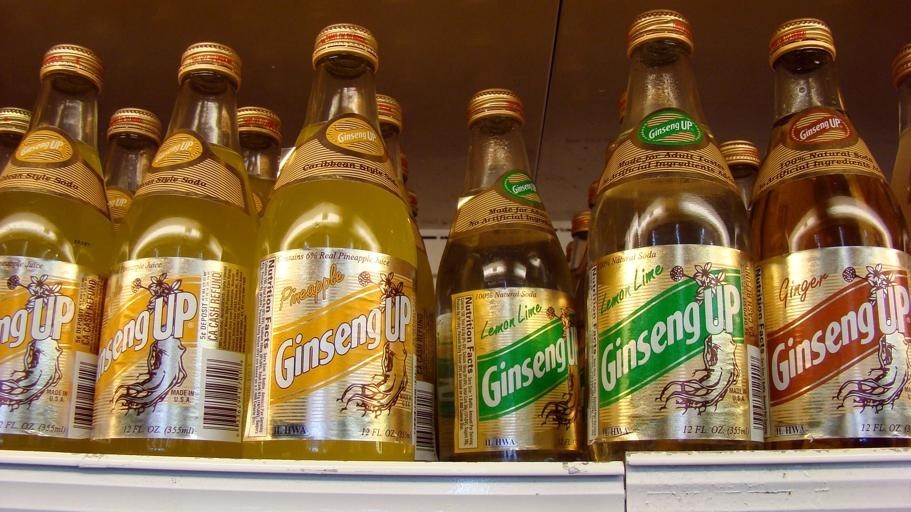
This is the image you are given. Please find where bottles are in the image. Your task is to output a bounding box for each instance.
[91,42,257,456]
[2,105,29,174]
[100,105,164,240]
[750,17,910,450]
[231,103,284,218]
[439,88,584,460]
[253,24,437,462]
[567,11,766,453]
[2,43,111,453]
